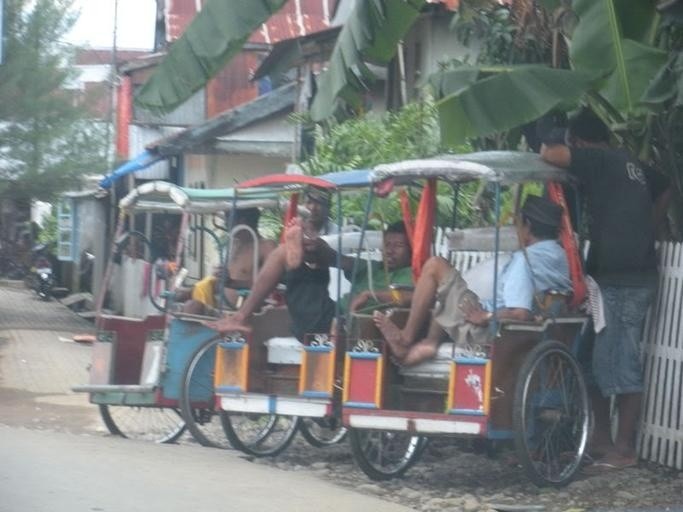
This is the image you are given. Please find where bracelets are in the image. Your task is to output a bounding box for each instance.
[487,311,493,321]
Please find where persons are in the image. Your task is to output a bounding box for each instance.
[173,209,277,315]
[539,109,672,476]
[373,192,572,368]
[294,185,338,237]
[202,216,436,344]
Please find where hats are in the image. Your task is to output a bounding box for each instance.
[521,194,565,227]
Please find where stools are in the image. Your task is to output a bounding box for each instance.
[398,343,462,412]
[264,336,307,394]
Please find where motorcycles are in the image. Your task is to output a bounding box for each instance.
[30,257,54,300]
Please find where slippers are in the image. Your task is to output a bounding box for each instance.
[580,451,638,475]
